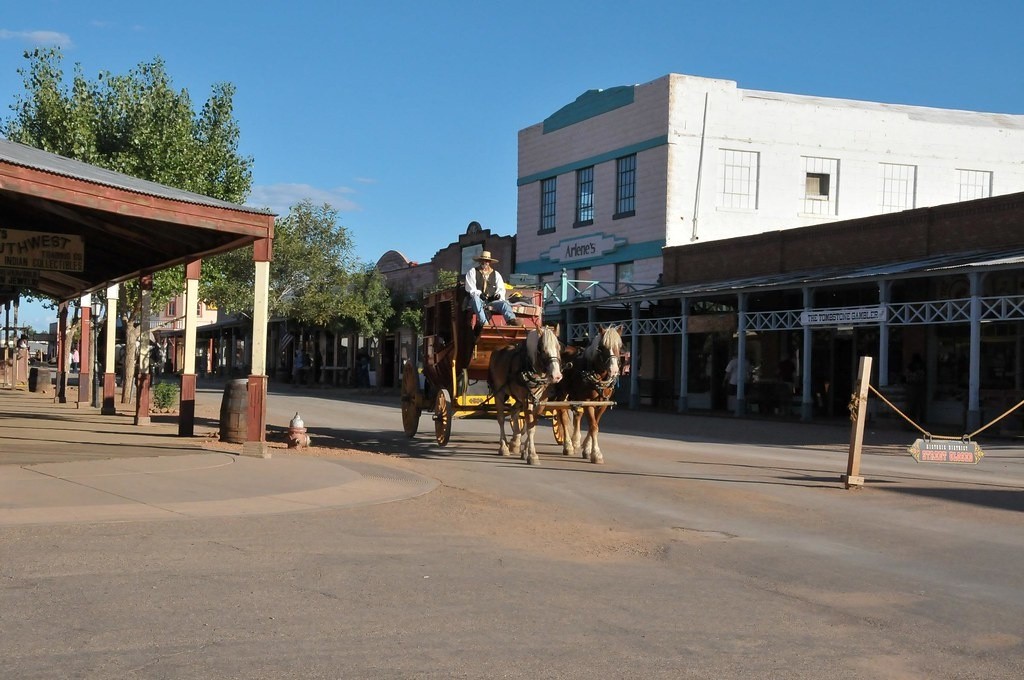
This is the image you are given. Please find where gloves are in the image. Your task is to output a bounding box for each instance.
[480,292,489,300]
[492,294,500,299]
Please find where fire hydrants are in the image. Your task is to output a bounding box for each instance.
[286,411,311,451]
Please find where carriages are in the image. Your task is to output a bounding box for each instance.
[97,319,168,388]
[400,272,631,466]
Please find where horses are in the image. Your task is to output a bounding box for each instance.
[488,322,563,465]
[545,323,623,464]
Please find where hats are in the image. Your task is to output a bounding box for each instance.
[472,251,499,264]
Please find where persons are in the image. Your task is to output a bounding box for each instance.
[466,251,524,326]
[290,348,306,387]
[355,347,372,389]
[68,347,79,374]
[36,350,40,361]
[722,353,750,417]
[302,353,313,385]
[17,334,30,348]
[312,350,323,384]
[164,358,173,373]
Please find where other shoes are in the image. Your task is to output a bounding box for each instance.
[506,318,523,327]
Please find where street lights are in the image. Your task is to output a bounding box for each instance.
[91,297,103,408]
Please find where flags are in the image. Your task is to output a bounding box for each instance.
[278,328,293,353]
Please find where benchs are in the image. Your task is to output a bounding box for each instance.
[462,290,542,326]
